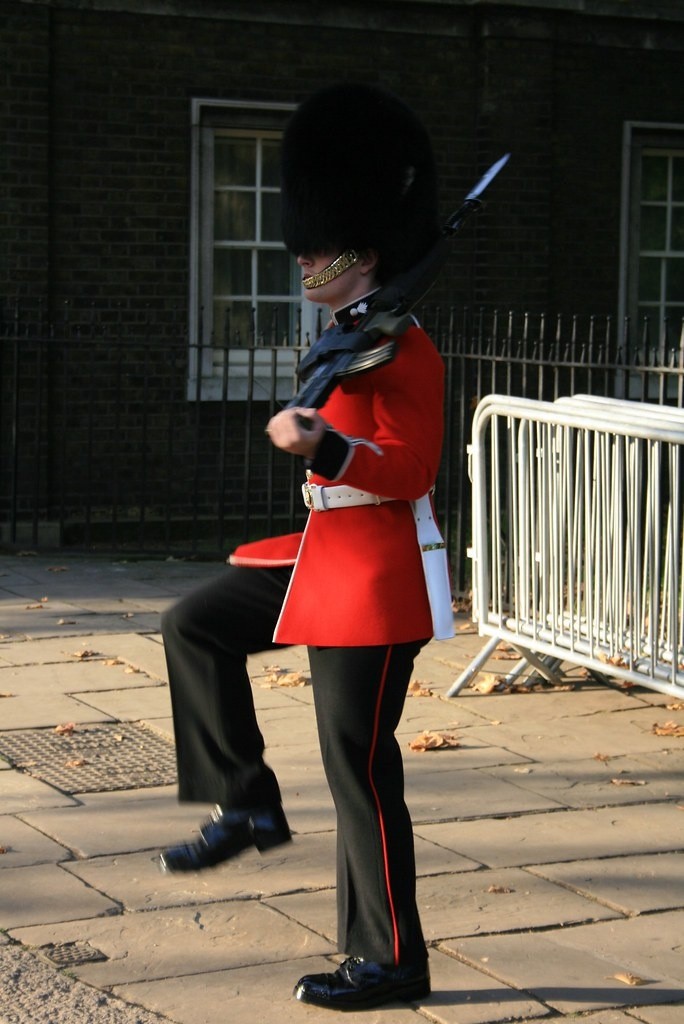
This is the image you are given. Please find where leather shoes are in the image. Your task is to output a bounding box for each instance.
[157,801,294,876]
[291,956,433,1011]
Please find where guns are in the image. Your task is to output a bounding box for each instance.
[280,150,514,432]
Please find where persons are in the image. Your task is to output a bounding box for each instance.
[160,239,456,1012]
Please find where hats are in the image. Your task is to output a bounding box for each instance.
[279,80,446,254]
